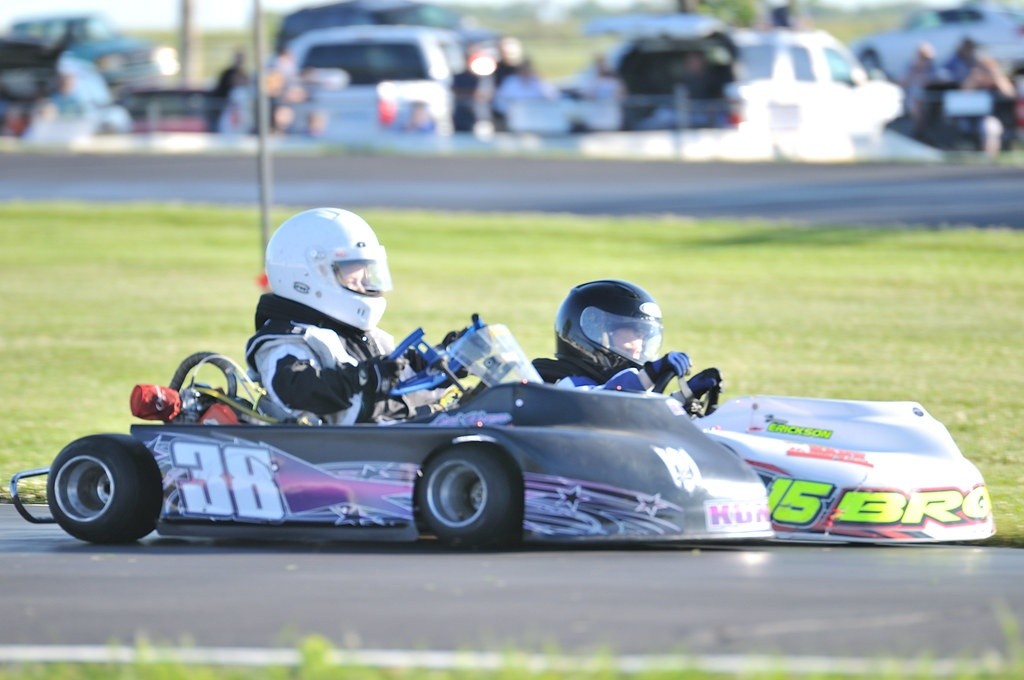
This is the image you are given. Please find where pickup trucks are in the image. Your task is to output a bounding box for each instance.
[275,28,455,148]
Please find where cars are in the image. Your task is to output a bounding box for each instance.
[848,0,1024,86]
[0,8,177,111]
[270,0,531,92]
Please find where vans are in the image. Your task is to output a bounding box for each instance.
[581,12,885,148]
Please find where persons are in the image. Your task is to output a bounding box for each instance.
[20,75,97,146]
[907,39,1024,152]
[450,34,719,136]
[214,48,327,138]
[533,280,721,406]
[394,102,436,137]
[246,207,493,429]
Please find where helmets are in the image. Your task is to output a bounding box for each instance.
[555,279,664,382]
[265,207,391,332]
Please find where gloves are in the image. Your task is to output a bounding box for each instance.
[687,368,722,400]
[645,352,692,383]
[357,355,399,396]
[443,323,492,353]
